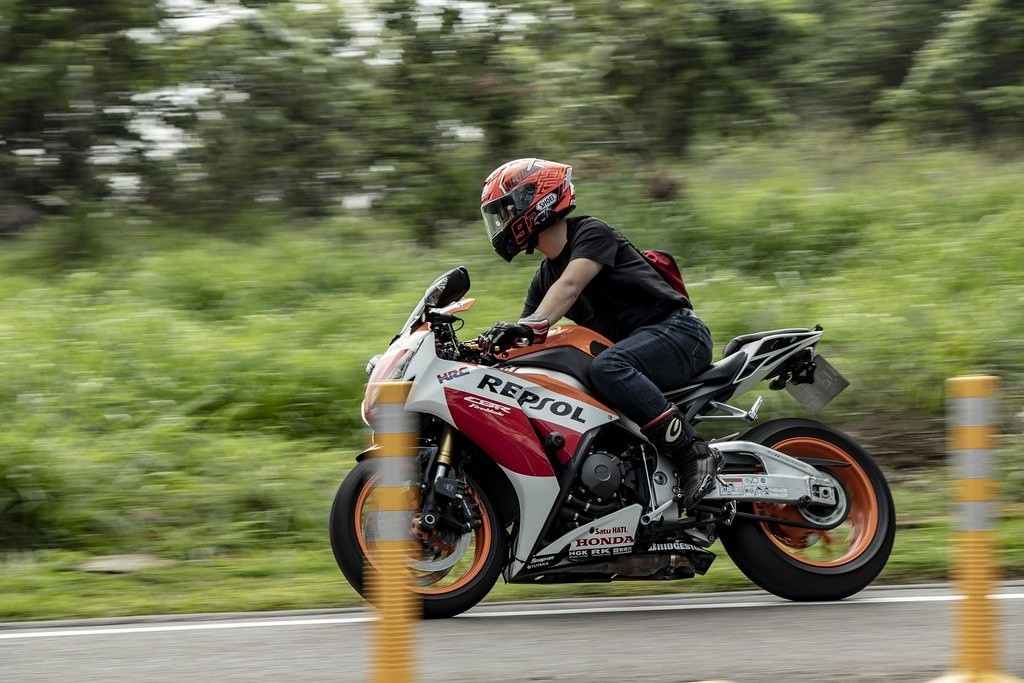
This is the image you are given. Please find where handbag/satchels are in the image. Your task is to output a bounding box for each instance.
[641,249,689,299]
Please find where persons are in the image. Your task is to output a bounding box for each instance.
[454,159,723,509]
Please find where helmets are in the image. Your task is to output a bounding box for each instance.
[481,158,576,263]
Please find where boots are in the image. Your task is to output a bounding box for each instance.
[640,402,721,510]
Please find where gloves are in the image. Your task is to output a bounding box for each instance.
[478,318,549,356]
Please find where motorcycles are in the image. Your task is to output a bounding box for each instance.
[329,266,895,620]
